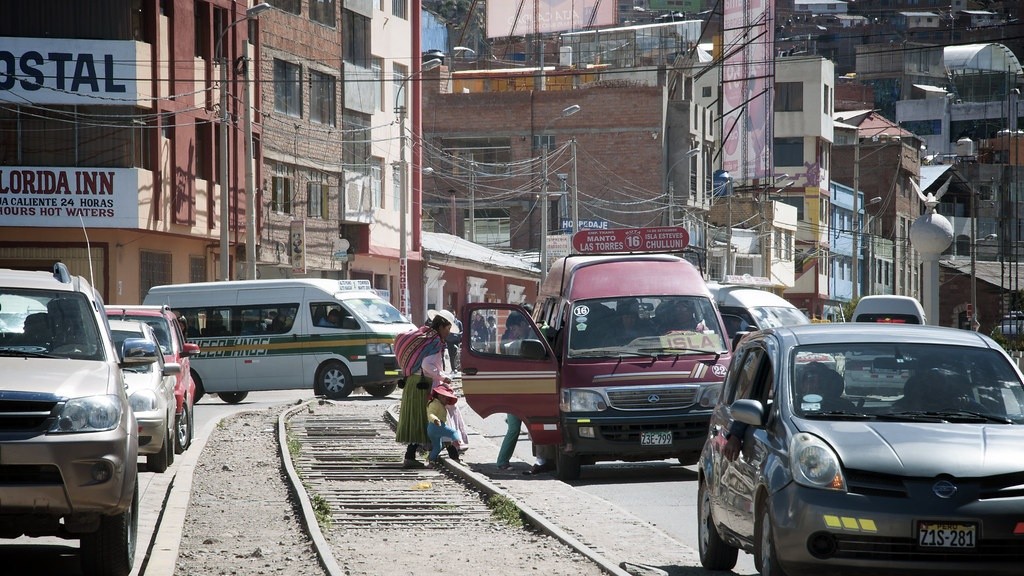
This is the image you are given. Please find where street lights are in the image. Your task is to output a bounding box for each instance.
[971,232,999,331]
[851,196,884,302]
[215,1,271,279]
[666,147,702,226]
[759,173,789,277]
[394,57,445,322]
[542,104,582,290]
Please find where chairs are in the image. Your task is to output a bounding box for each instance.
[793,370,858,419]
[3,313,63,355]
[882,369,982,416]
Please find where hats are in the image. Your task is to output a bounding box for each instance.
[427,308,460,334]
[432,384,457,406]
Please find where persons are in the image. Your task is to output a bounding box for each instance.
[425,382,470,468]
[425,309,463,374]
[178,316,188,335]
[187,311,292,336]
[497,304,560,475]
[396,310,458,466]
[319,309,340,326]
[722,362,855,462]
[472,313,497,353]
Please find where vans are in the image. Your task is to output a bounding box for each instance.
[707,275,811,354]
[0,293,49,333]
[462,252,743,478]
[851,295,928,325]
[140,277,421,405]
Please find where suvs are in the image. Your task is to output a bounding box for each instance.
[0,260,142,576]
[104,301,204,450]
[991,310,1024,351]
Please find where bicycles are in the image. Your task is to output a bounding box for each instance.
[454,335,491,371]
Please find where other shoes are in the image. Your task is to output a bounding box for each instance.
[453,458,468,466]
[523,464,547,475]
[457,446,468,454]
[404,458,424,467]
[498,465,513,470]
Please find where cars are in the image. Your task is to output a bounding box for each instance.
[697,322,1024,576]
[108,320,178,473]
